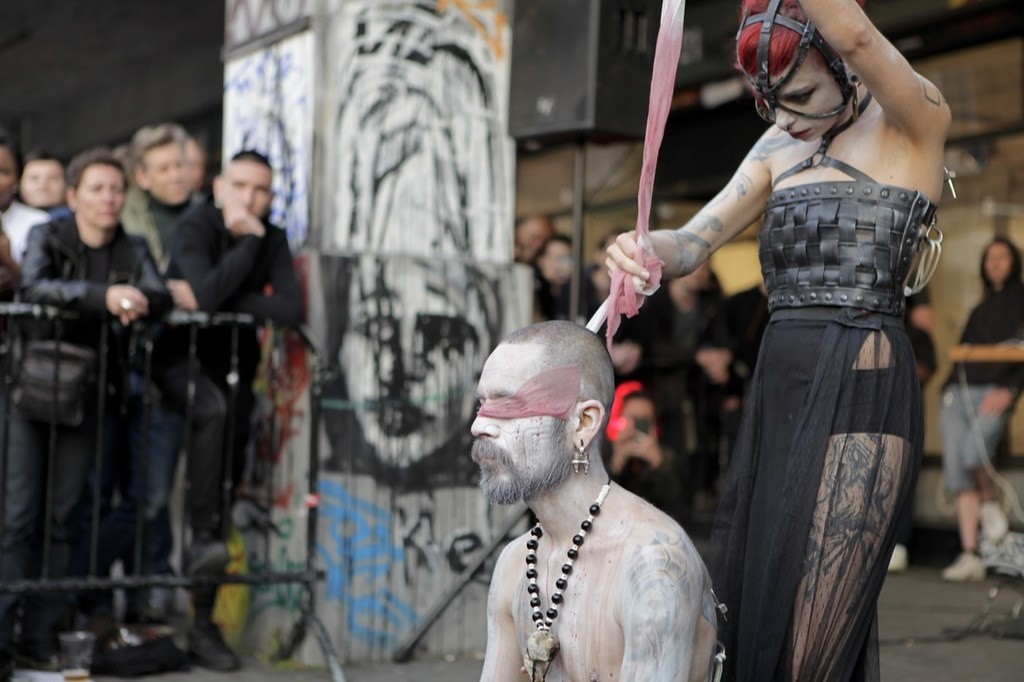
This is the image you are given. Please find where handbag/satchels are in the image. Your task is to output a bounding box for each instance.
[9,340,97,426]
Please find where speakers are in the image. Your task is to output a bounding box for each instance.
[508,0,662,151]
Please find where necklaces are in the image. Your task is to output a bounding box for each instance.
[523,473,614,682]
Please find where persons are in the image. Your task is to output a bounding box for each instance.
[884,220,1024,581]
[514,196,773,559]
[0,102,319,682]
[451,320,717,682]
[603,0,950,681]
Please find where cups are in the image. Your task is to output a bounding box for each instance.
[58,631,96,682]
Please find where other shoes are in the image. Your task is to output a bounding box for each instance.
[187,623,241,672]
[887,544,909,572]
[91,643,163,677]
[181,540,231,574]
[941,552,986,581]
[978,499,1008,545]
[12,636,67,672]
[130,634,190,670]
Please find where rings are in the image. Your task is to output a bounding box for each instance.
[119,296,137,315]
